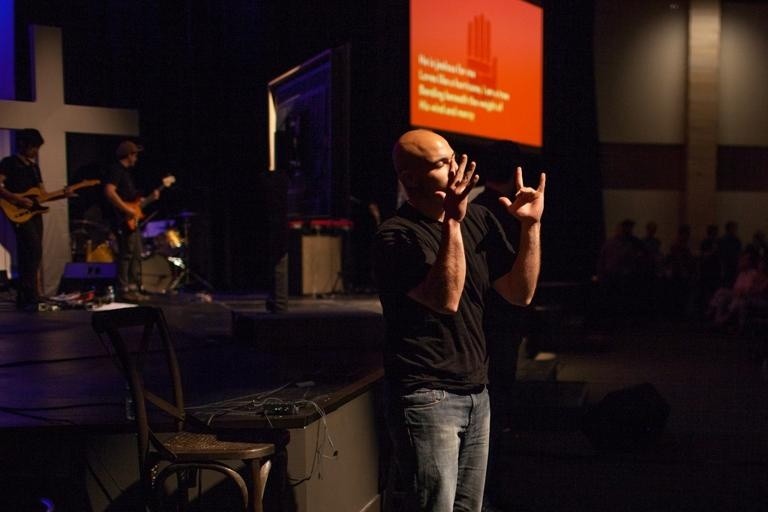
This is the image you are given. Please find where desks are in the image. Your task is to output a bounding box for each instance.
[183,347,385,512]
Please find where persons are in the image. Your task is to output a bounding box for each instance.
[378,129,547,512]
[103,141,159,301]
[0,128,78,309]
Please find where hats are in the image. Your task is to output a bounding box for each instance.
[116,140,142,155]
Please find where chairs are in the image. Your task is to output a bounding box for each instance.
[91,304,276,512]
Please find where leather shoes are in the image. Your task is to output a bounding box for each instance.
[122,289,148,303]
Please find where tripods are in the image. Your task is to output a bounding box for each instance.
[171,222,213,292]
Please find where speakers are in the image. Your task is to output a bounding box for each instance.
[64,262,118,294]
